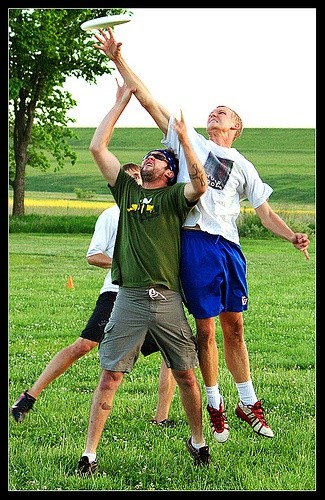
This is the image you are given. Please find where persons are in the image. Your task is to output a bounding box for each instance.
[76,76,211,477]
[12,162,179,430]
[91,26,311,443]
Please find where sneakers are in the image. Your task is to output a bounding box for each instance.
[186,437,212,466]
[235,398,275,438]
[68,456,98,479]
[206,396,230,443]
[10,390,36,422]
[151,418,176,428]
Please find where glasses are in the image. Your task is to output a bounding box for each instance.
[143,153,169,164]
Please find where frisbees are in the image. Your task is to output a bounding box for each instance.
[80,14,132,31]
